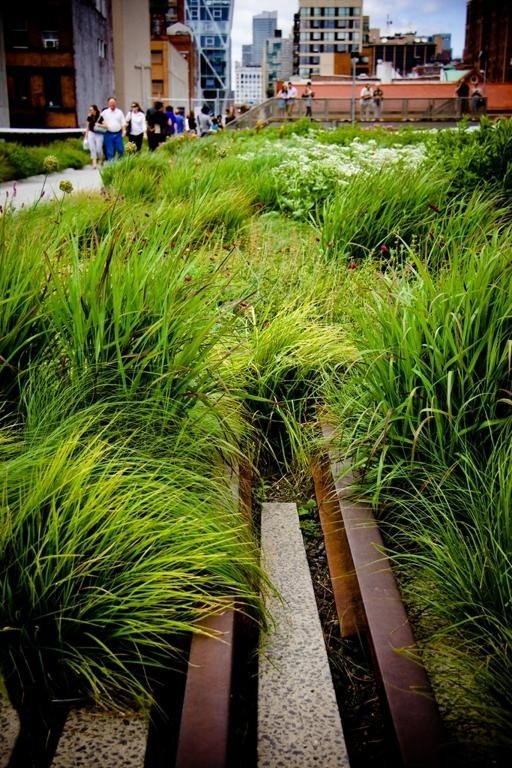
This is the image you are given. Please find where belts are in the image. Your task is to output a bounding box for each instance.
[107,130,121,135]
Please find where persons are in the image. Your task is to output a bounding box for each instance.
[286,82,298,121]
[303,81,317,120]
[371,82,385,123]
[276,83,288,120]
[81,94,273,168]
[471,81,482,123]
[360,82,376,121]
[456,75,470,119]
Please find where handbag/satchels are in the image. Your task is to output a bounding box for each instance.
[301,94,308,100]
[126,119,131,136]
[83,132,89,151]
[93,115,108,133]
